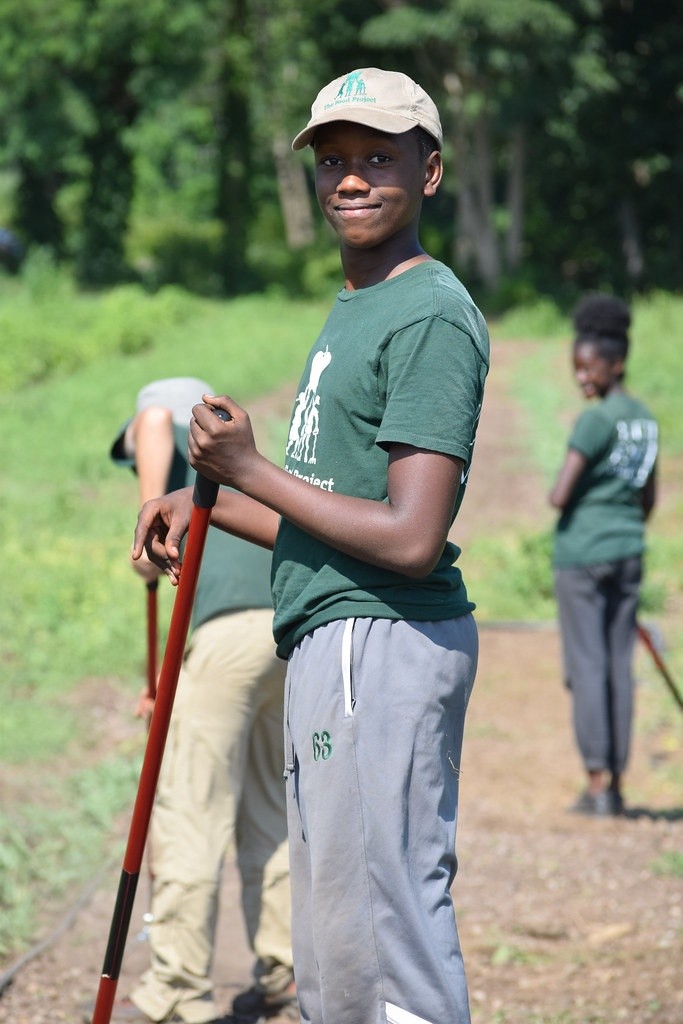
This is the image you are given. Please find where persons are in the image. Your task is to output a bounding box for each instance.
[129,64,493,1024]
[550,294,663,817]
[107,377,290,1024]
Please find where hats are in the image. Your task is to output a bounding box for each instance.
[292,66,442,152]
[137,378,215,427]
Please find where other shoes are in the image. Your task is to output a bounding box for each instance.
[571,789,622,816]
[83,996,182,1024]
[248,961,300,1006]
